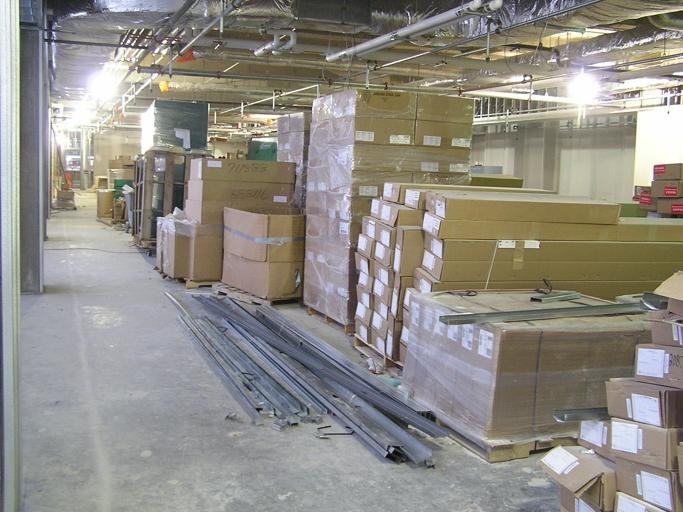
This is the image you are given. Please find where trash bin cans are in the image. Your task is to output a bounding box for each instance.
[122,190,134,233]
